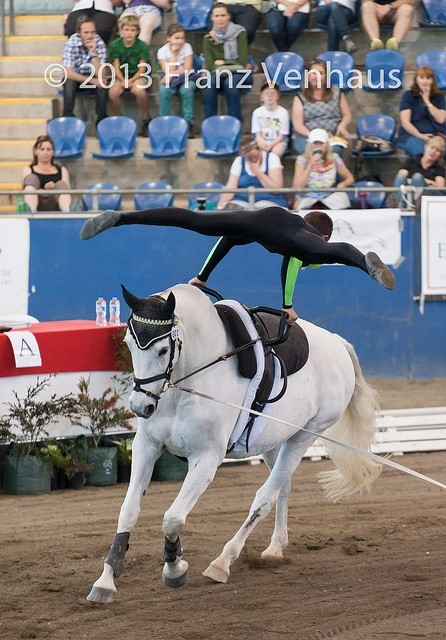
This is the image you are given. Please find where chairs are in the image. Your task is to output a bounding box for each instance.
[316,51,355,93]
[396,126,410,148]
[59,77,98,97]
[192,53,201,74]
[92,115,138,158]
[264,51,305,93]
[253,120,293,157]
[363,49,405,92]
[418,0,445,31]
[173,0,216,31]
[82,182,122,211]
[351,113,395,156]
[197,115,241,158]
[353,181,386,208]
[143,115,189,159]
[47,117,87,158]
[415,50,446,90]
[109,59,150,95]
[188,181,225,206]
[134,181,173,210]
[220,54,254,93]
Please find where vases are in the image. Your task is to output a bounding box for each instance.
[56,468,87,488]
[77,447,118,485]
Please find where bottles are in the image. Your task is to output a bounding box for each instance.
[109,292,122,325]
[95,293,106,326]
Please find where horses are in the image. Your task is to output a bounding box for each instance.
[82,283,387,607]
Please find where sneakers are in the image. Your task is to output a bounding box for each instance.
[79,209,121,240]
[365,252,396,290]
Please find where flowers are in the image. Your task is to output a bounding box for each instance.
[40,439,97,480]
[67,372,135,449]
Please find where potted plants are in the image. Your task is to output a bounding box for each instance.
[111,326,188,481]
[0,374,85,495]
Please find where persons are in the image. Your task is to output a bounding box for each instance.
[217,0,261,45]
[251,83,290,159]
[386,136,446,208]
[202,3,253,124]
[216,134,290,208]
[106,15,153,138]
[291,59,353,160]
[294,128,352,210]
[21,135,72,213]
[63,1,123,44]
[264,1,310,51]
[79,207,396,327]
[361,0,415,51]
[60,14,107,128]
[315,1,359,59]
[117,0,173,48]
[157,24,201,138]
[400,67,446,159]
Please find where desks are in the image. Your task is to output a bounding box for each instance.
[0,318,143,447]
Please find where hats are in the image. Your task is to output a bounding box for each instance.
[308,128,328,144]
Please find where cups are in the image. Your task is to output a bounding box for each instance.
[15,195,26,212]
[311,148,324,164]
[196,197,207,210]
[359,191,367,208]
